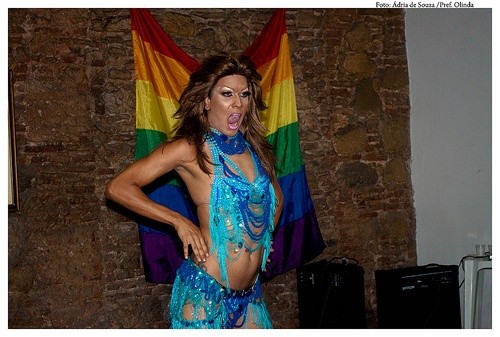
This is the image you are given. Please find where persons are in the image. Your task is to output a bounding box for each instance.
[104,51,283,328]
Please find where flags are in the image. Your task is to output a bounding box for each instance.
[130,8,327,284]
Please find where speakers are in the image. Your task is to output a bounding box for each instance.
[374,263,461,329]
[296,261,367,329]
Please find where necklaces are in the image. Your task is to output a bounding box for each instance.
[203,128,259,183]
